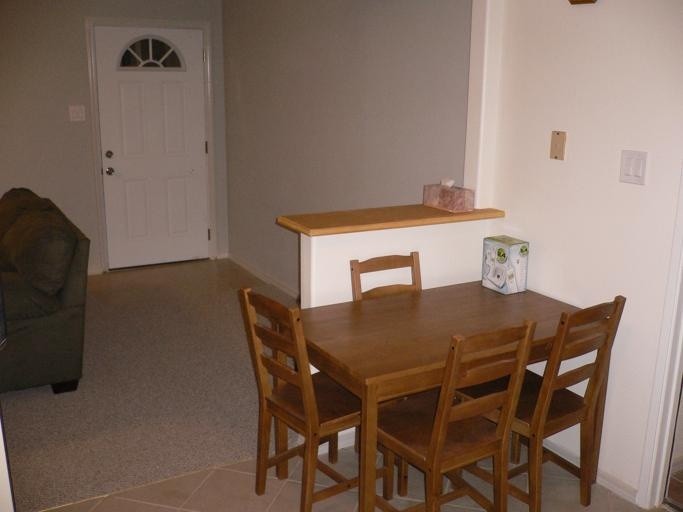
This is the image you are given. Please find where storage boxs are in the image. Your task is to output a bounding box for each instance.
[482,235,530,296]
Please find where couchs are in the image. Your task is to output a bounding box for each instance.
[0,198,90,394]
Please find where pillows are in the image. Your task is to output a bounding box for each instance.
[0,188,41,271]
[3,211,76,296]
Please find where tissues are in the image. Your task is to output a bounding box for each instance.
[423,180,475,210]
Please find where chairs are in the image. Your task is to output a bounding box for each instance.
[349,251,423,454]
[373,320,538,512]
[238,287,397,512]
[455,295,626,512]
